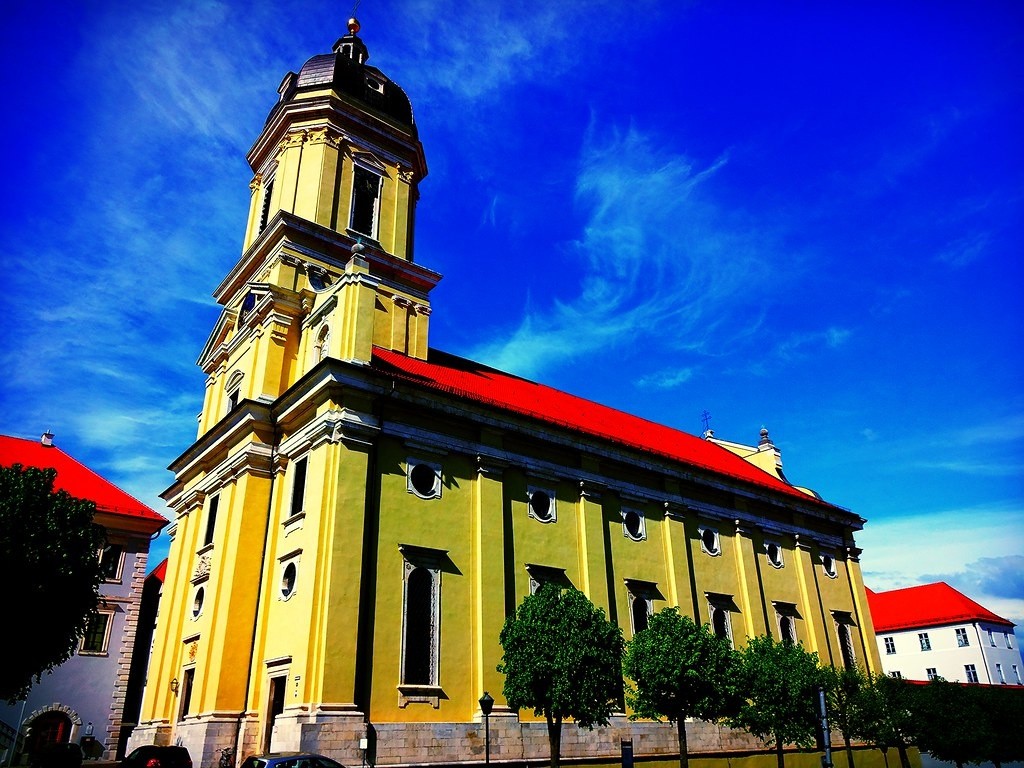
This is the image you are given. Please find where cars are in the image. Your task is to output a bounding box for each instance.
[238,752,345,768]
[118,745,193,768]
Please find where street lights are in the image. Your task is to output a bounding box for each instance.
[479,692,494,767]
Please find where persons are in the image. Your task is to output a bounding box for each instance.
[363,720,378,768]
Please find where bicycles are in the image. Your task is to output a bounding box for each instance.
[216,747,234,768]
